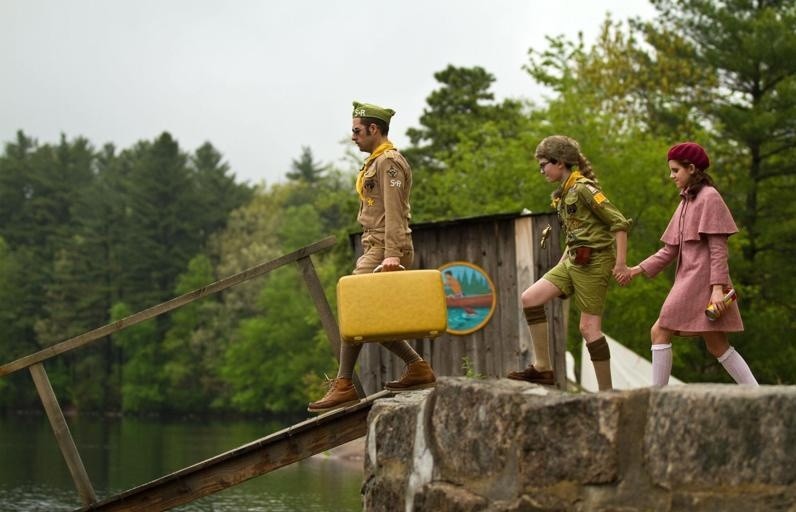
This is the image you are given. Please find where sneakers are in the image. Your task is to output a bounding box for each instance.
[507,365,554,384]
[384,361,437,391]
[308,377,360,412]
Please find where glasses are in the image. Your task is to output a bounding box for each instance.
[540,161,550,167]
[352,127,362,135]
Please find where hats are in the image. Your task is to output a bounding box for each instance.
[668,143,709,168]
[353,101,395,123]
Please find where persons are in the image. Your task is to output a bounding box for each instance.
[611,141,763,388]
[441,269,475,317]
[304,98,438,413]
[506,133,632,399]
